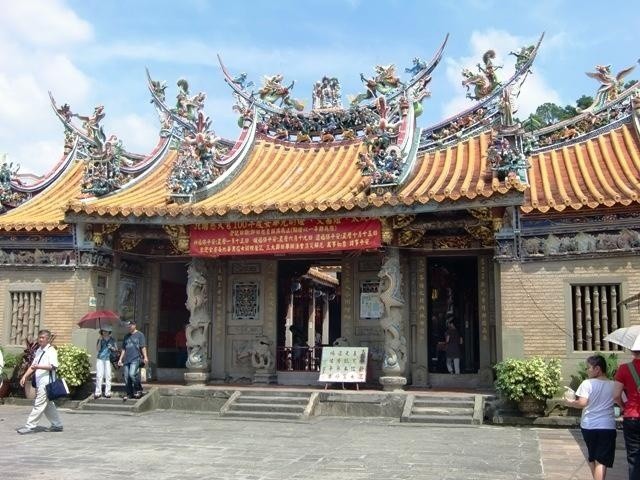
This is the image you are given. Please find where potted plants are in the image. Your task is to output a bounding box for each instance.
[0,343,92,400]
[490,356,568,418]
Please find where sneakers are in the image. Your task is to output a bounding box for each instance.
[45,425,64,432]
[17,427,35,434]
[94,391,142,400]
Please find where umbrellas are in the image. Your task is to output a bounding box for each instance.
[76,309,121,341]
[601,324,640,351]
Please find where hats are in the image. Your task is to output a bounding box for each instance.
[98,324,113,336]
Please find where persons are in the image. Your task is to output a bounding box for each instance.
[93,323,118,399]
[564,354,618,480]
[613,351,639,480]
[173,318,189,368]
[443,318,463,374]
[278,325,336,371]
[118,319,149,401]
[18,330,63,434]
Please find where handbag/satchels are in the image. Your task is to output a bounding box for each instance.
[45,364,70,401]
[139,354,145,367]
[31,371,36,388]
[109,350,122,370]
